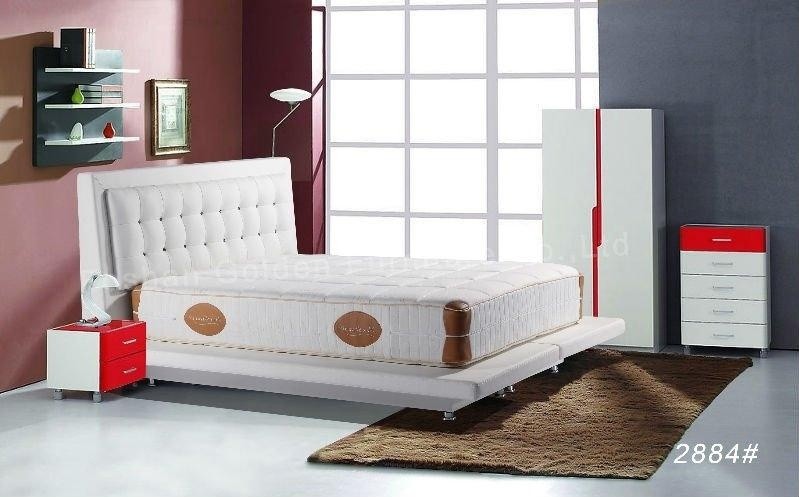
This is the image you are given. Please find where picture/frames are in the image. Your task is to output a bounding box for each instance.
[149,77,192,156]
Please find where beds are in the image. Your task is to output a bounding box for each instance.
[75,156,625,421]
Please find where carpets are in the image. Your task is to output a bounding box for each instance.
[306,347,753,481]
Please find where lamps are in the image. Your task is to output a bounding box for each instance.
[75,269,119,326]
[269,87,312,156]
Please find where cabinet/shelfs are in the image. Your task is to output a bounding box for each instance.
[541,106,665,352]
[679,223,772,358]
[44,65,140,146]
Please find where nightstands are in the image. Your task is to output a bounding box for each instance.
[45,320,147,403]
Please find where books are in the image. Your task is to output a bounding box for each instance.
[102,97,123,104]
[102,84,125,92]
[101,92,123,97]
[93,28,96,69]
[77,84,102,92]
[83,98,101,104]
[58,26,89,70]
[82,91,101,98]
[88,28,93,69]
